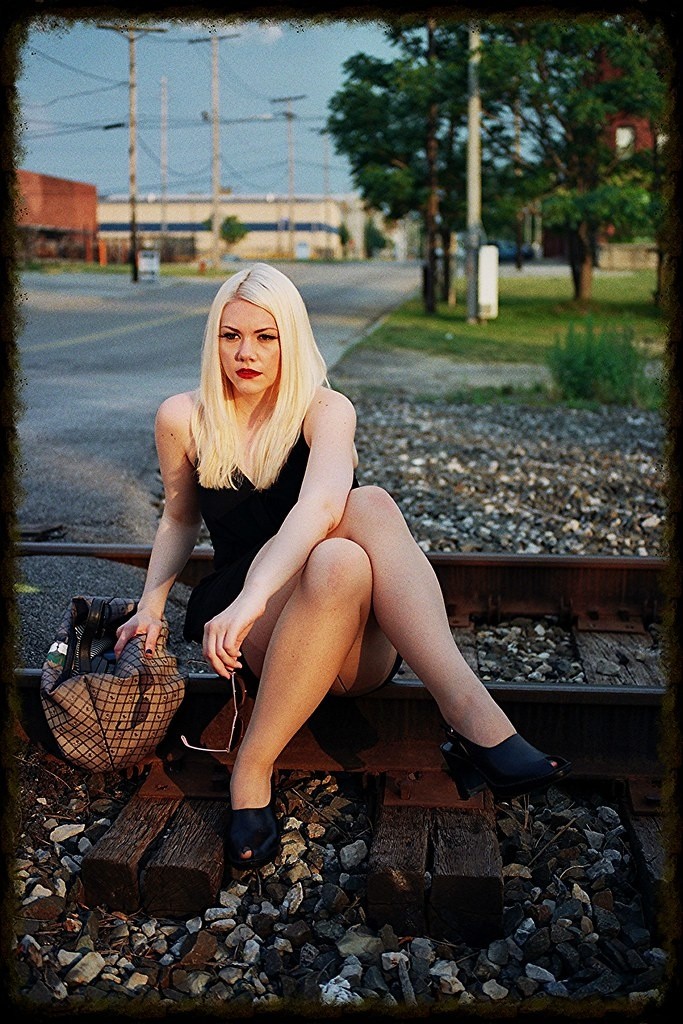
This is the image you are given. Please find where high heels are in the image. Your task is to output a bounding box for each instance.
[231,796,277,867]
[440,726,568,804]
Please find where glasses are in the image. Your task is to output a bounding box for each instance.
[182,659,245,754]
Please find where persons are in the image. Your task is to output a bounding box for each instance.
[113,264,568,867]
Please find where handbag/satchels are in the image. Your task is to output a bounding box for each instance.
[42,597,185,771]
